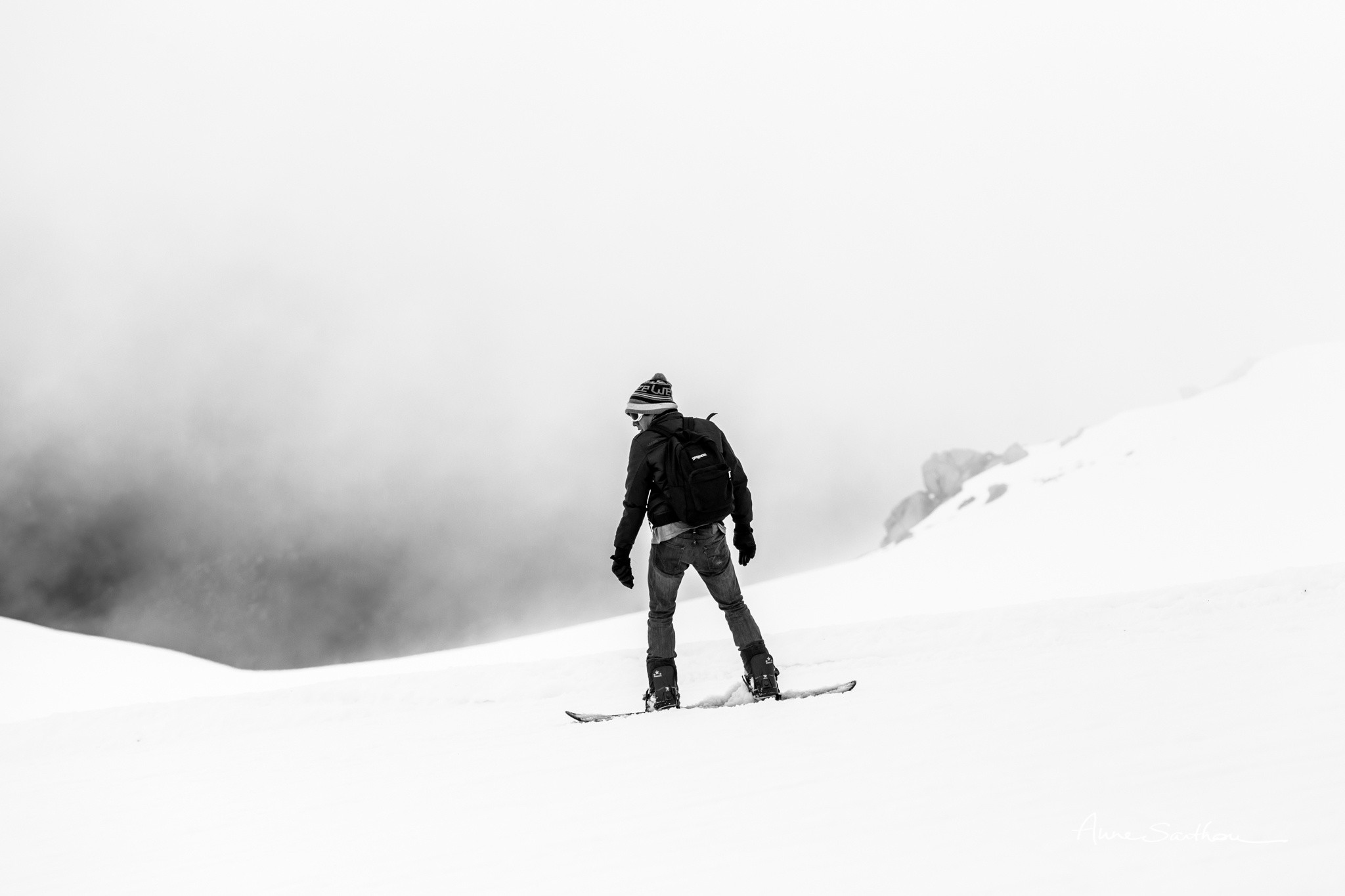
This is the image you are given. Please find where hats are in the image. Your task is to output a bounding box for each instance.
[624,372,677,414]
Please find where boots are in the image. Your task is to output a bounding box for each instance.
[738,644,782,701]
[645,659,680,711]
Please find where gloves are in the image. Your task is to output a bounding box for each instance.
[733,527,756,566]
[610,550,634,589]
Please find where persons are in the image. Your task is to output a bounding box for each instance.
[612,372,781,712]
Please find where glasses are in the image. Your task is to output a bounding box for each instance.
[630,414,653,421]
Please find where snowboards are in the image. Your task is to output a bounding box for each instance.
[564,656,857,724]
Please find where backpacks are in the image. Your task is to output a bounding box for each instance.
[645,415,735,527]
